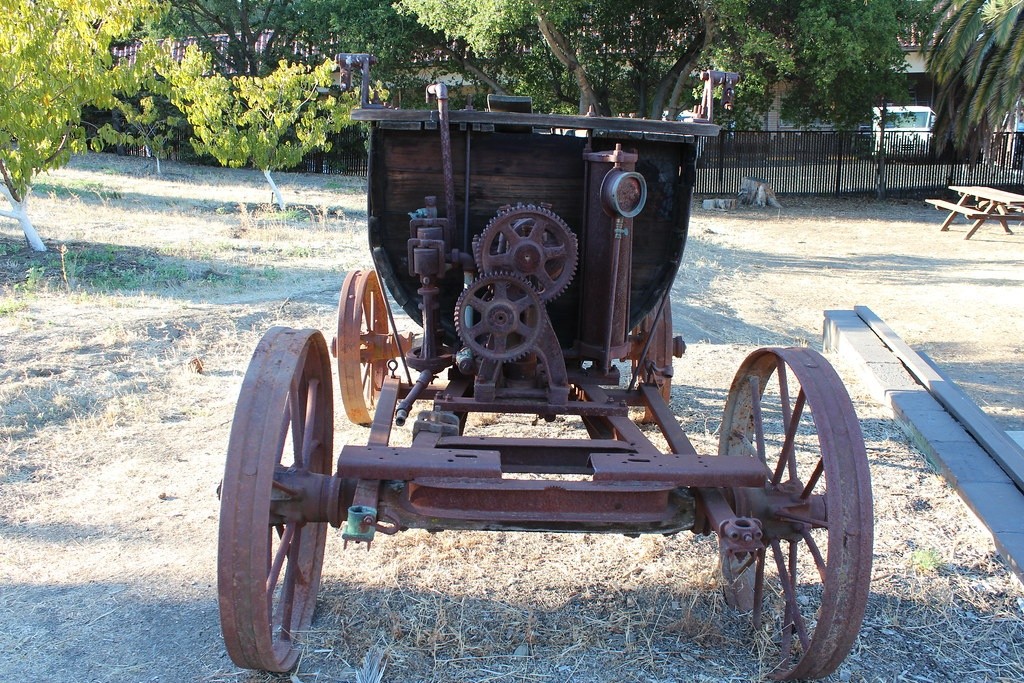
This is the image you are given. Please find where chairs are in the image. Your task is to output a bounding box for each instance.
[925,198,1024,218]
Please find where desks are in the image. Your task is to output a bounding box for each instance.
[939,186,1024,240]
